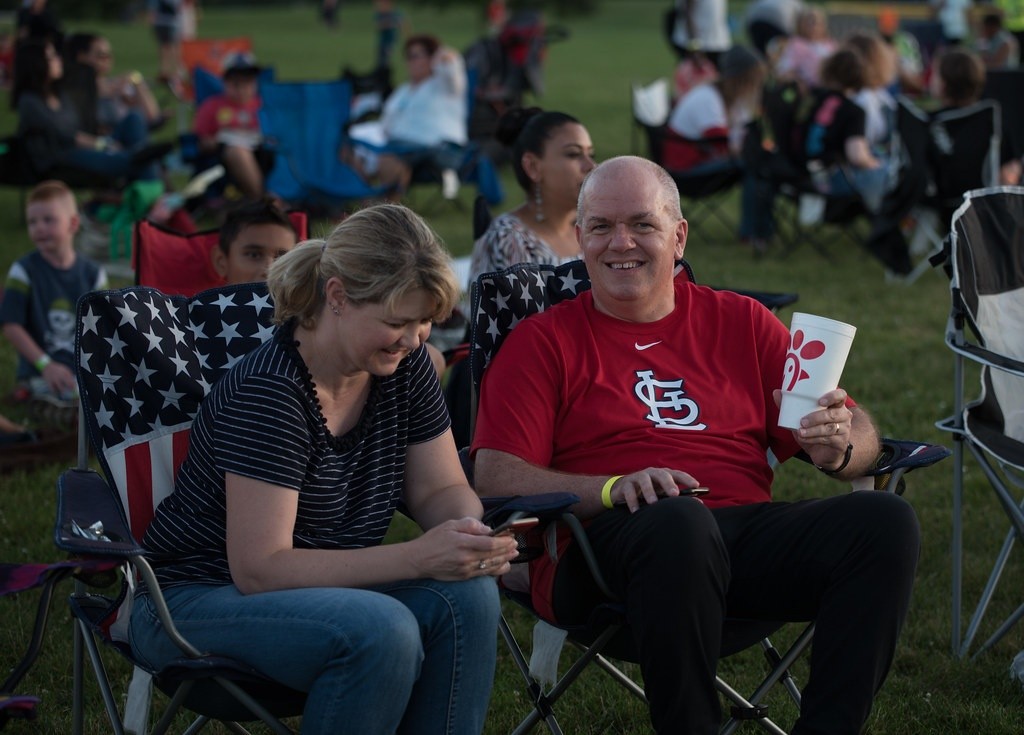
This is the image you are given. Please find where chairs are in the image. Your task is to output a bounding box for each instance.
[0,14,1024,735]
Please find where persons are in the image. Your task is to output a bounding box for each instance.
[123,204,521,734]
[0,182,112,429]
[470,155,919,733]
[0,1,1024,286]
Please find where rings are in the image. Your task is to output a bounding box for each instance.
[835,422,840,433]
[478,560,487,570]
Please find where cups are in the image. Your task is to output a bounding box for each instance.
[777,312,859,430]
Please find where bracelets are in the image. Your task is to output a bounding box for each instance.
[814,444,854,474]
[600,475,624,508]
[34,356,49,371]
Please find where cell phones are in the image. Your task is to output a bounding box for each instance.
[487,518,540,538]
